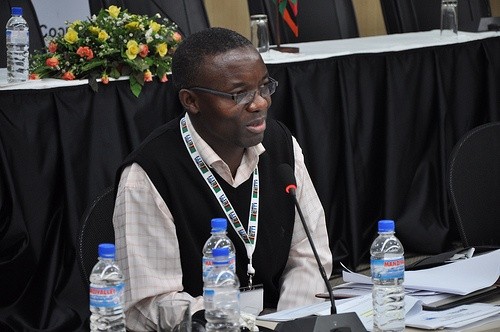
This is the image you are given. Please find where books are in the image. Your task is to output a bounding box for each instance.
[315,284,500,311]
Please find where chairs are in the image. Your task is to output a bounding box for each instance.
[78,186,116,289]
[449,122,500,249]
[89,0,209,39]
[248,0,361,43]
[0,0,47,67]
[381,0,492,34]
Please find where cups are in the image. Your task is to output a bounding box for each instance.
[157,299,191,332]
[249,14,270,59]
[440,0,459,43]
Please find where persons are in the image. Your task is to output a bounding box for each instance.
[113,28,332,332]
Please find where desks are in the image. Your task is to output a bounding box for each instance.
[0,28,500,332]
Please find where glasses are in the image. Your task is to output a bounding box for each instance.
[190,77,279,105]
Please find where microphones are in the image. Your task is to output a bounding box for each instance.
[271,164,367,332]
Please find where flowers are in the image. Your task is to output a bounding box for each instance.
[28,6,180,97]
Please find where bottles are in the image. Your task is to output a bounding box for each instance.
[202,218,241,332]
[5,6,29,84]
[369,220,406,332]
[89,243,127,332]
